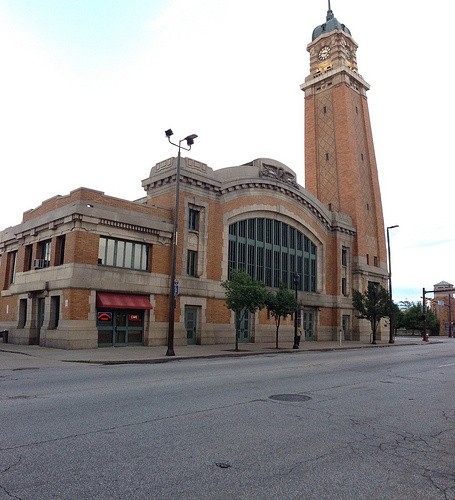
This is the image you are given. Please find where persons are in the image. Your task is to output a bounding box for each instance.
[297,325,303,346]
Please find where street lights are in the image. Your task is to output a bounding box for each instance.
[387,225,399,343]
[293,272,300,348]
[165,129,198,356]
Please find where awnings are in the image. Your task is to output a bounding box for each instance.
[95,292,154,310]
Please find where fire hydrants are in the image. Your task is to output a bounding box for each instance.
[425,333,429,342]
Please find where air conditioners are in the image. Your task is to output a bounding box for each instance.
[33,259,43,269]
